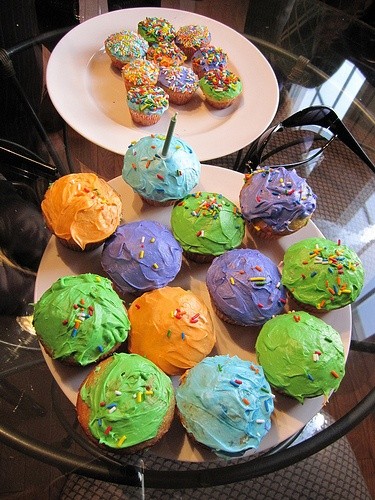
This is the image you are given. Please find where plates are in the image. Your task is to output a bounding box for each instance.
[33,163,353,463]
[45,7,280,163]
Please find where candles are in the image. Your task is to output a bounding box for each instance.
[162,113,178,157]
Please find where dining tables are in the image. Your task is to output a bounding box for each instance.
[0,25,375,488]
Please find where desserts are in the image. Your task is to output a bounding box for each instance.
[32,134,365,460]
[105,17,242,126]
